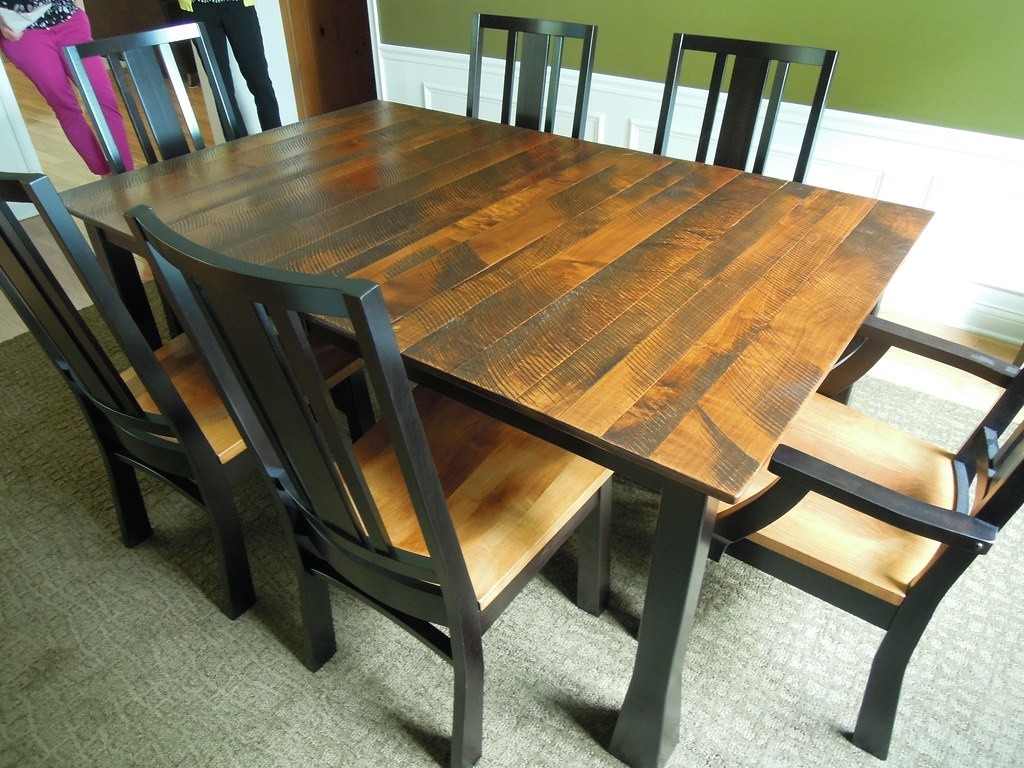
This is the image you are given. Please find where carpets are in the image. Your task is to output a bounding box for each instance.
[0,273,1024,768]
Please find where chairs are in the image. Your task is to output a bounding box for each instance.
[57,21,247,176]
[464,12,599,140]
[0,170,378,622]
[707,293,1024,761]
[124,202,617,768]
[651,31,839,185]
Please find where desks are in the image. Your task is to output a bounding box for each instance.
[56,95,943,768]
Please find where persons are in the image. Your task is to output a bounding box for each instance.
[175,0,281,141]
[0,0,135,180]
